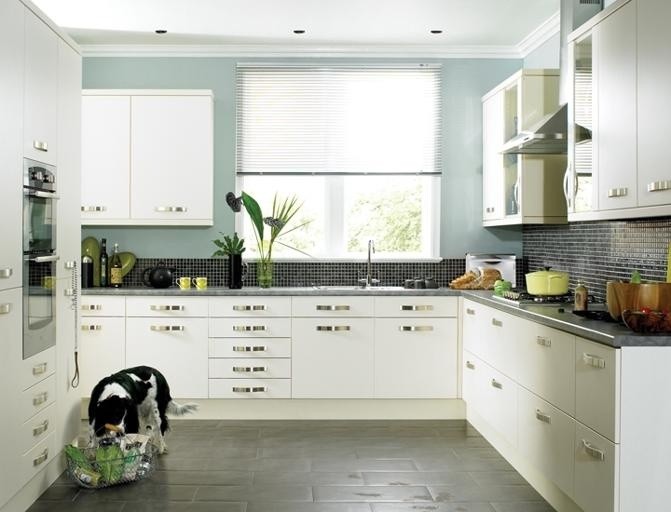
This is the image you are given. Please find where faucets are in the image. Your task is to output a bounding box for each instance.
[365,238,377,287]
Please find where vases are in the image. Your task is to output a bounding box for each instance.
[255,239,274,288]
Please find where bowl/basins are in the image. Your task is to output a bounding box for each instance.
[621,310,667,333]
[606,280,670,323]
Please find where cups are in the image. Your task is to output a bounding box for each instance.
[175,277,191,290]
[44,277,52,289]
[404,277,436,289]
[192,277,207,291]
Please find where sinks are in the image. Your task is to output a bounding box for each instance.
[312,285,405,292]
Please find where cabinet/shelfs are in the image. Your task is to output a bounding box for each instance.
[131,88,215,227]
[81,87,131,228]
[566,0,671,224]
[481,68,569,229]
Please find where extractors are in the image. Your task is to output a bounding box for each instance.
[502,1,604,154]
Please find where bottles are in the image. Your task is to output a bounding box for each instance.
[511,184,517,214]
[574,279,588,311]
[81,238,123,288]
[65,439,152,486]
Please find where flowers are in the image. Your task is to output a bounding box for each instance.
[225,187,315,283]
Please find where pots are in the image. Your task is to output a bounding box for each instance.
[525,266,569,296]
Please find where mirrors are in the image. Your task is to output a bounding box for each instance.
[572,32,597,214]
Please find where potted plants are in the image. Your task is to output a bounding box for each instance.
[210,231,245,289]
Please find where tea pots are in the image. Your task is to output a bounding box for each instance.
[142,263,175,289]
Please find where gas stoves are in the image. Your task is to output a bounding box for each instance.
[492,290,607,308]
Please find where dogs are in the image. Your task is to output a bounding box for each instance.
[87,365,202,454]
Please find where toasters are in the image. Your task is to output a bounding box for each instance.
[465,252,516,289]
